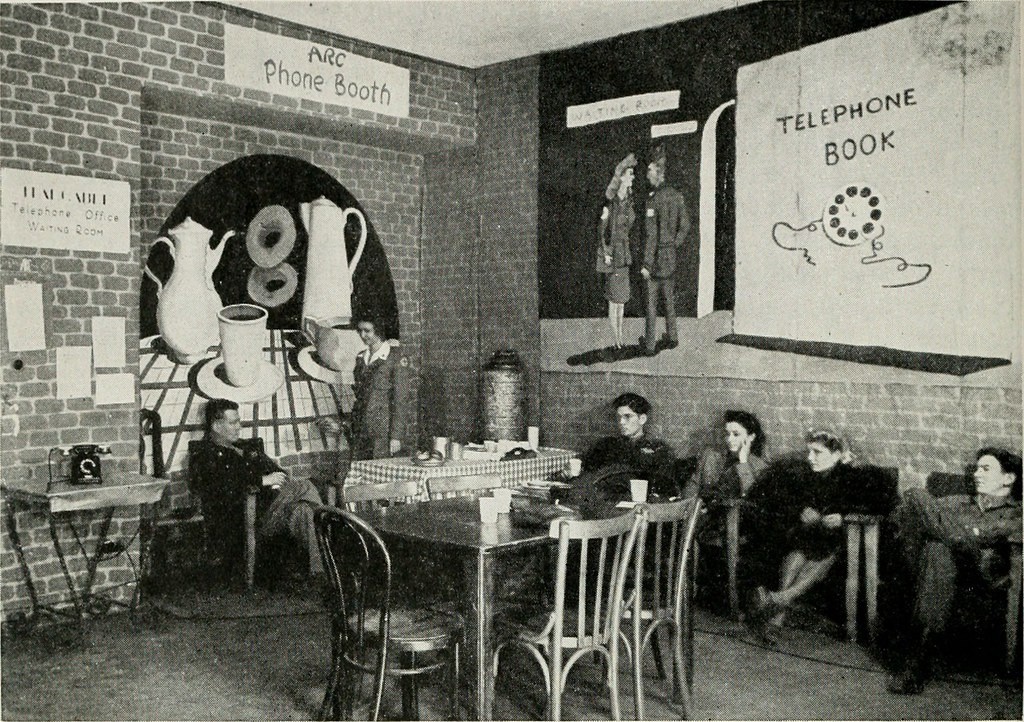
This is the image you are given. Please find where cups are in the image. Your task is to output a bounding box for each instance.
[479,497,498,524]
[301,314,369,372]
[569,458,582,477]
[493,489,511,514]
[630,479,648,507]
[216,304,268,386]
[484,439,528,462]
[527,426,539,451]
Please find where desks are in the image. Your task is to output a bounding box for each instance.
[342,443,583,503]
[354,496,632,720]
[0,473,173,655]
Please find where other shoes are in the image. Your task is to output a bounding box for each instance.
[762,621,783,650]
[886,669,925,695]
[324,577,361,598]
[752,585,777,622]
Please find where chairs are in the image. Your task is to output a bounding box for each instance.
[567,492,702,719]
[187,438,279,589]
[486,503,647,722]
[309,505,466,722]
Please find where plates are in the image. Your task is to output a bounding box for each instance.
[297,345,360,384]
[196,357,286,403]
[521,481,572,493]
[410,458,448,467]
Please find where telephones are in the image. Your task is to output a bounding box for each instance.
[47,445,111,486]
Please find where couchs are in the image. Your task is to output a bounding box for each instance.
[660,447,1023,677]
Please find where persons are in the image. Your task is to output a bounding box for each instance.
[888,447,1022,695]
[199,400,342,585]
[350,312,406,551]
[502,393,680,620]
[752,428,863,648]
[681,410,767,600]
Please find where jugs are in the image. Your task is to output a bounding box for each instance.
[144,216,236,365]
[299,194,368,330]
[432,432,462,461]
